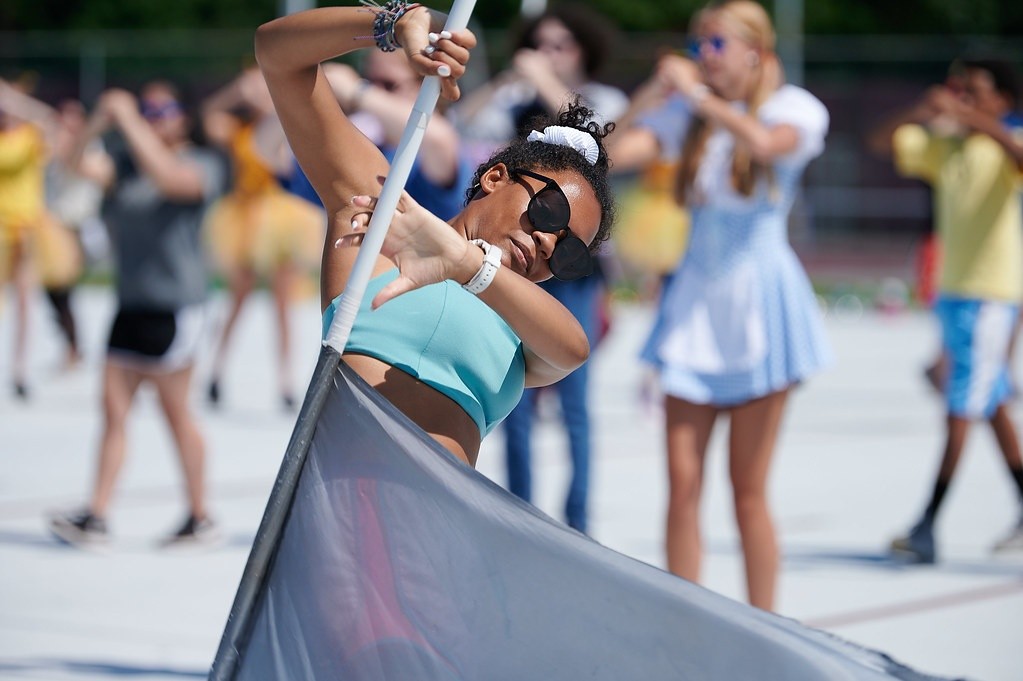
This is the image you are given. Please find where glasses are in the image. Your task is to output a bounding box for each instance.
[689,39,723,54]
[474,168,593,282]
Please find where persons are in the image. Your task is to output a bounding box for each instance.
[874,59,1023,563]
[321,0,829,609]
[256,0,616,472]
[0,66,325,547]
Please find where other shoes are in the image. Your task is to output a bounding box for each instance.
[174,516,213,538]
[46,511,108,549]
[890,526,936,563]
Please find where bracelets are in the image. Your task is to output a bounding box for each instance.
[685,86,709,110]
[353,0,419,52]
[460,238,502,293]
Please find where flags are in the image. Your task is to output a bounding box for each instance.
[236,365,959,681]
